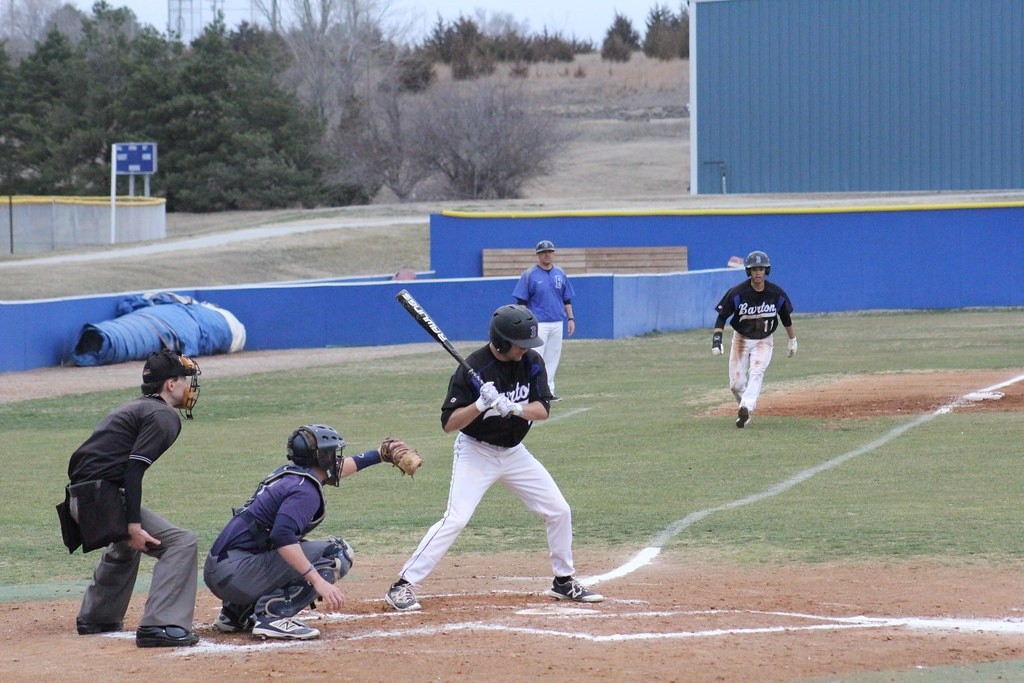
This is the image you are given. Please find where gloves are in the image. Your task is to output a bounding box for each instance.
[712,332,724,356]
[476,382,499,412]
[787,337,797,358]
[491,395,521,418]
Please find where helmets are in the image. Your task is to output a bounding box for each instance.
[744,251,771,277]
[535,240,555,253]
[489,304,544,353]
[286,425,347,488]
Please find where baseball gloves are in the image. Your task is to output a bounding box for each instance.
[381,437,423,475]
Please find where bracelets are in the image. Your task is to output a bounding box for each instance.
[568,318,573,321]
[303,565,313,576]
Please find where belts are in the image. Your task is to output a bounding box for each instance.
[67,482,97,496]
[216,555,228,562]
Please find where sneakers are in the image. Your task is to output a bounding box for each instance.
[76,617,123,634]
[252,614,320,640]
[549,578,603,602]
[212,608,257,632]
[136,626,199,647]
[385,582,422,612]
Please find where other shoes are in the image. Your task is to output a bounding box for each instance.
[549,395,562,402]
[735,406,749,428]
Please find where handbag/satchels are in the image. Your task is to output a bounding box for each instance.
[55,484,81,554]
[74,484,132,552]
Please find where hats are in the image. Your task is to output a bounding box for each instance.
[142,352,202,382]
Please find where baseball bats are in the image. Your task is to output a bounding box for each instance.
[395,289,513,418]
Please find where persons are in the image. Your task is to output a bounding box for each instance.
[384,304,603,611]
[203,423,423,642]
[712,250,797,428]
[513,240,575,403]
[55,349,202,648]
